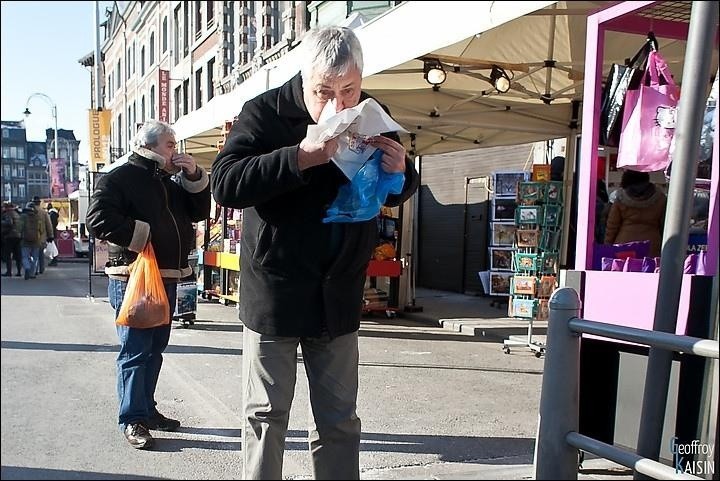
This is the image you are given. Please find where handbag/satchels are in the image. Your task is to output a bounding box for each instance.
[615,50,681,174]
[1,215,14,235]
[598,42,651,150]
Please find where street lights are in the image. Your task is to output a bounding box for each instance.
[23,92,58,158]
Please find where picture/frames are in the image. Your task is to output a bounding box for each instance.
[489,171,565,322]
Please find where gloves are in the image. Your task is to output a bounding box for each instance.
[46,237,54,242]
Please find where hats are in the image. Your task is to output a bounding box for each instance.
[31,195,42,202]
[46,203,53,209]
[25,202,35,209]
[1,202,9,207]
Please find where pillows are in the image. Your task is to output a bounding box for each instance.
[592,239,698,274]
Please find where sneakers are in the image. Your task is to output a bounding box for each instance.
[124,419,155,449]
[142,408,181,432]
[1,261,58,281]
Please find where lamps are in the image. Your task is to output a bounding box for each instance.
[423,57,447,86]
[490,65,511,94]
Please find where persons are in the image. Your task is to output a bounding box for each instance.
[530,155,668,258]
[84,117,211,452]
[1,195,61,280]
[213,25,424,481]
